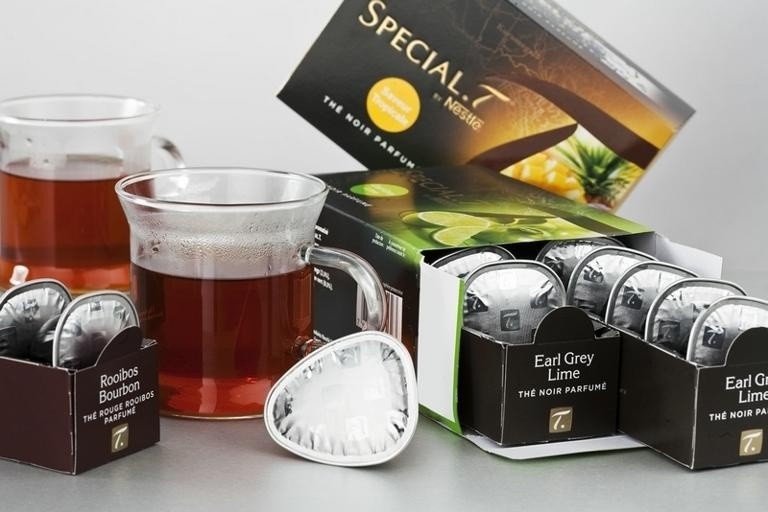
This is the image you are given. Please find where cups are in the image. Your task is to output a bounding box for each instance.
[118,167,387,420]
[1,92,183,308]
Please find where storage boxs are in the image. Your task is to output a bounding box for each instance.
[311,164,764,471]
